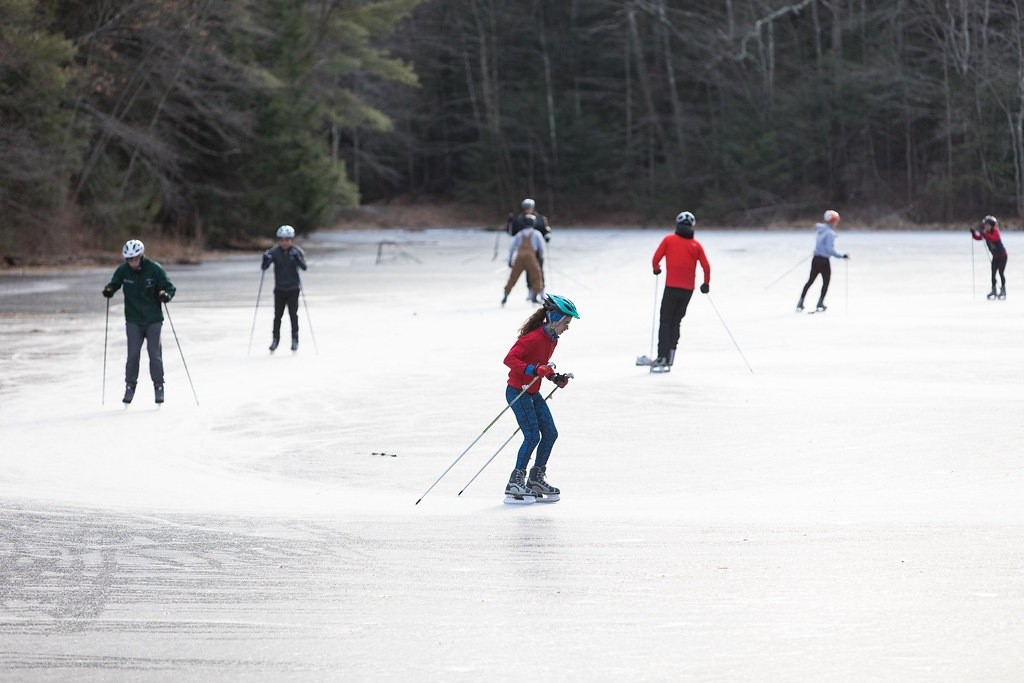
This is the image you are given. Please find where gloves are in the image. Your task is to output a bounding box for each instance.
[103,285,113,297]
[536,364,551,378]
[264,251,273,262]
[554,375,568,388]
[159,293,171,301]
[700,283,709,293]
[290,248,298,259]
[653,269,661,275]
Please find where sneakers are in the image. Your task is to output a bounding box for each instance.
[291,340,298,348]
[524,465,561,503]
[154,386,164,406]
[123,386,136,403]
[988,286,1007,300]
[501,293,545,308]
[504,468,537,503]
[647,347,674,372]
[270,340,280,352]
[796,303,805,311]
[817,302,827,311]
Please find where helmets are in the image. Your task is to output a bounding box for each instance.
[123,240,145,258]
[276,225,294,239]
[824,210,840,223]
[675,211,696,228]
[522,198,536,227]
[543,292,582,320]
[984,216,998,227]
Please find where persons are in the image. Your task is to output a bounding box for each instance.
[507,199,552,300]
[501,215,545,303]
[970,215,1007,298]
[652,212,710,365]
[504,294,580,504]
[796,210,848,310]
[103,240,176,405]
[261,226,308,352]
